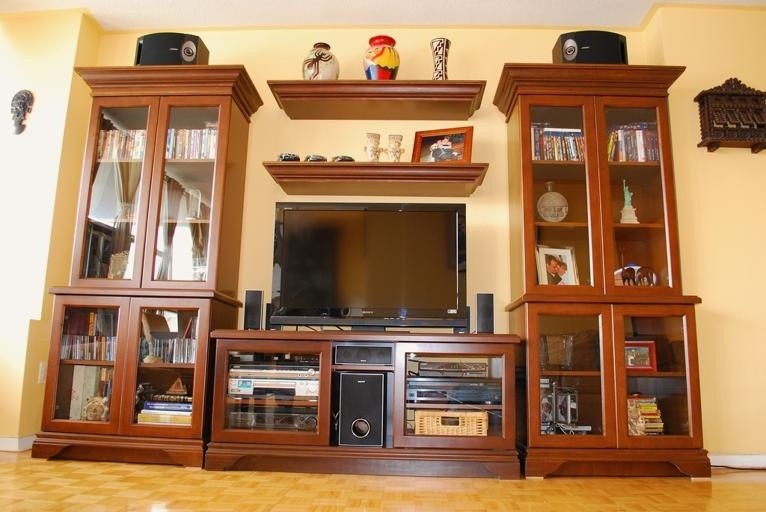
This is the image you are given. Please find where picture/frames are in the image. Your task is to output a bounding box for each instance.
[624,340,657,372]
[536,243,580,287]
[410,127,473,164]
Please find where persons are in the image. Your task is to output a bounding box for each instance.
[545,253,572,286]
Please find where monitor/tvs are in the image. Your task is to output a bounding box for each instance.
[269,201,467,334]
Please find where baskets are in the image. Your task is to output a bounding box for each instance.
[413,409,489,437]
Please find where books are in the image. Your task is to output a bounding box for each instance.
[69,364,114,422]
[60,309,117,362]
[137,394,194,426]
[164,127,219,160]
[530,122,586,162]
[606,121,660,162]
[140,315,198,365]
[627,393,665,436]
[96,128,147,160]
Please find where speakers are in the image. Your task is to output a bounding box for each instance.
[338,372,386,447]
[243,290,264,331]
[476,293,495,334]
[552,30,628,64]
[134,31,210,66]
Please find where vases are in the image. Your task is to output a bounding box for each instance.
[430,38,451,81]
[362,37,399,81]
[303,42,339,80]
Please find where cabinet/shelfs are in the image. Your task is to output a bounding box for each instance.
[508,304,702,448]
[68,92,249,294]
[263,161,488,196]
[507,93,681,296]
[267,78,487,121]
[42,296,238,438]
[396,345,512,451]
[213,341,331,447]
